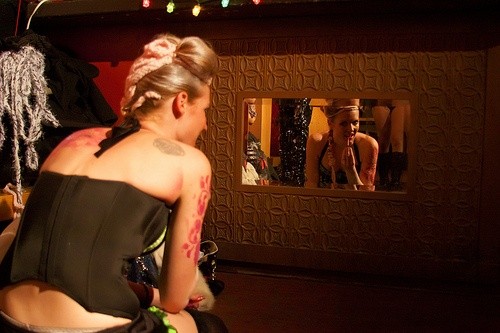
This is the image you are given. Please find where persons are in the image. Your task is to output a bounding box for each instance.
[0,31,231,333]
[302,97,379,191]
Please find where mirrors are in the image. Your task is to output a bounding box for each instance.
[235,90,416,200]
